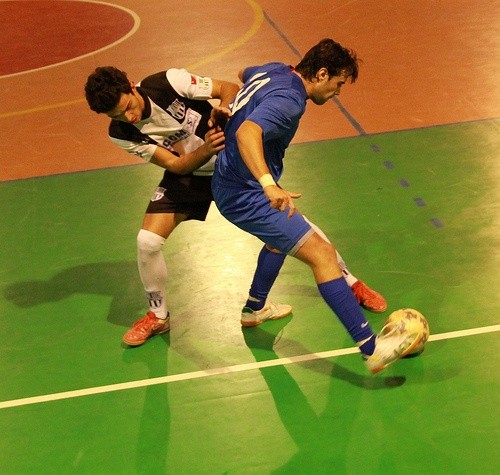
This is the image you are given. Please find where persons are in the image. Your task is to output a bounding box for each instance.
[211,37,422,373]
[84,65,387,346]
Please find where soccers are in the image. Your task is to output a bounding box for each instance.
[384,307,431,355]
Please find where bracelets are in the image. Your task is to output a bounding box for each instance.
[258,173,276,190]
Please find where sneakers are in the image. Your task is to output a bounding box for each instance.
[364,321,424,373]
[240,300,292,327]
[122,310,171,345]
[350,279,387,312]
